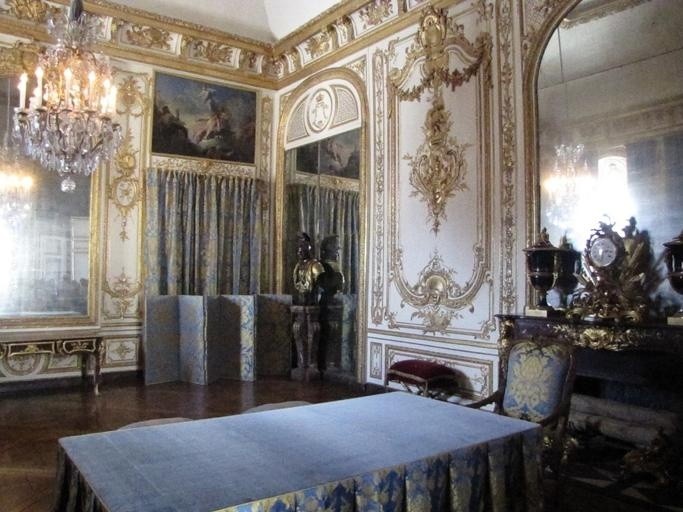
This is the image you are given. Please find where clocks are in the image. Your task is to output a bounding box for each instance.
[566,213,649,324]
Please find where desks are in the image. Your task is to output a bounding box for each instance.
[56,390,544,512]
[0,330,103,396]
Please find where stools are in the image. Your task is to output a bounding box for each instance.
[384,359,455,400]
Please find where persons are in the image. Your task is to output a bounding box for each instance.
[315,233,346,375]
[157,104,188,140]
[286,230,325,383]
[194,112,221,143]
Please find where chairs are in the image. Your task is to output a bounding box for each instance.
[461,336,577,512]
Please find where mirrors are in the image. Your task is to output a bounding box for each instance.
[1,46,112,330]
[525,0,681,327]
[280,128,365,385]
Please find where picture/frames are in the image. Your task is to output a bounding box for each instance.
[147,67,263,169]
[295,129,362,181]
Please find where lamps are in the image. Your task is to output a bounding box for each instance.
[523,226,563,318]
[542,27,588,218]
[12,0,122,193]
[2,77,36,233]
[662,229,683,325]
